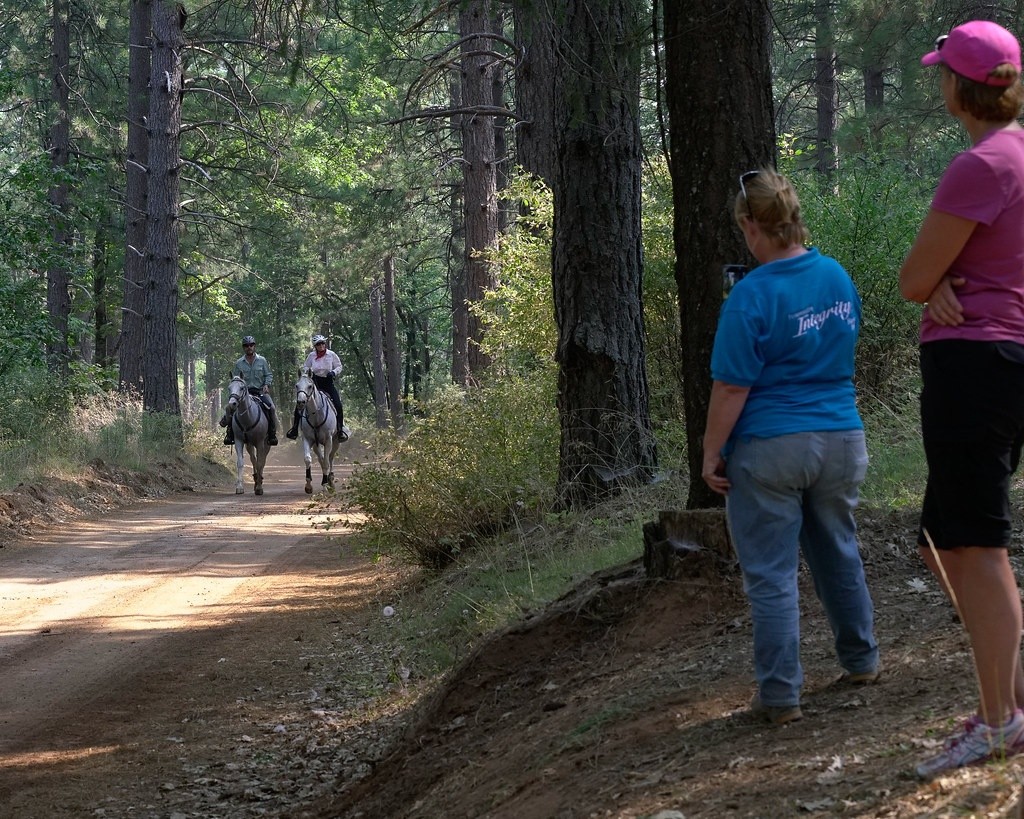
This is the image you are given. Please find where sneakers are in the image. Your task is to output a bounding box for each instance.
[914,707,1024,781]
[750,695,804,727]
[849,661,882,689]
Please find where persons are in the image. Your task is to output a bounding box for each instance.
[288,334,344,439]
[224,336,278,445]
[896,19,1024,779]
[701,167,882,724]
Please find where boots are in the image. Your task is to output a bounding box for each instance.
[224,407,235,445]
[337,409,345,441]
[287,405,304,438]
[268,407,278,444]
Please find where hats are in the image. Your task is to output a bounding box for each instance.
[921,20,1022,86]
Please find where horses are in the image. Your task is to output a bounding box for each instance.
[226,369,283,496]
[294,367,350,498]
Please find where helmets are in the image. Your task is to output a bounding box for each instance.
[312,334,326,345]
[242,336,256,345]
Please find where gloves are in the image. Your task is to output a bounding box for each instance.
[327,371,335,379]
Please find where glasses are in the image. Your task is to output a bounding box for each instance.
[739,171,781,223]
[316,342,325,346]
[934,34,960,80]
[243,343,255,347]
